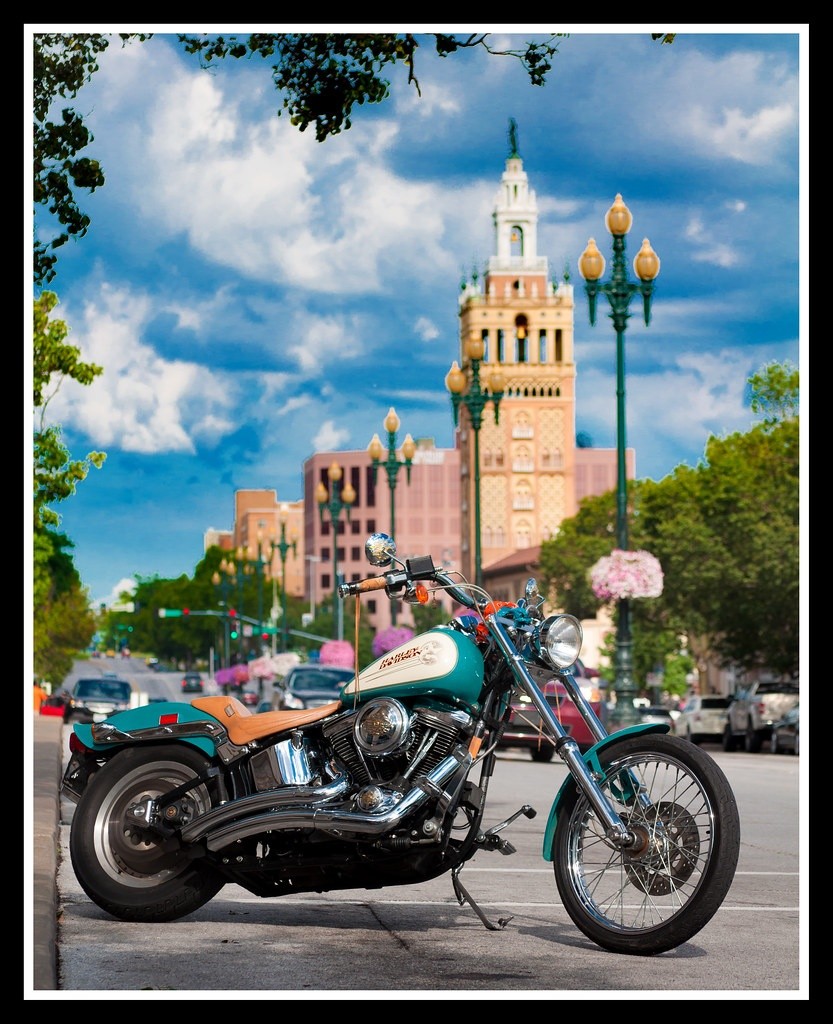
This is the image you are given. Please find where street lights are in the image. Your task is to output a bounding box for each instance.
[445,333,504,596]
[211,509,299,669]
[366,407,416,628]
[317,460,356,639]
[580,193,662,724]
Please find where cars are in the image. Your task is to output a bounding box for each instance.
[483,657,608,761]
[93,648,168,672]
[182,672,203,692]
[242,664,355,713]
[639,679,799,755]
[69,673,133,724]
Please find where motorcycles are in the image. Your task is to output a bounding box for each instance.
[70,534,741,957]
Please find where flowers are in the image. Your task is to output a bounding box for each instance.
[216,651,305,687]
[372,626,413,656]
[585,548,664,600]
